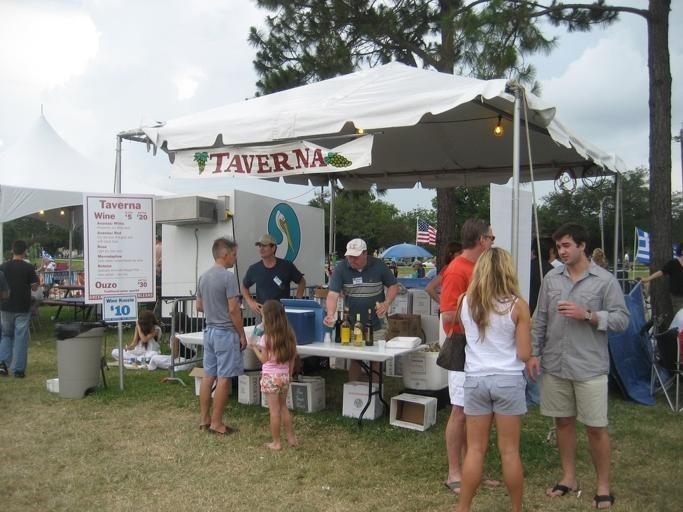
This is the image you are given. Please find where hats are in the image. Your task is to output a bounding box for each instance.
[344,238,368,256]
[255,234,276,245]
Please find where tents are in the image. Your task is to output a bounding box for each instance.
[117,62,631,399]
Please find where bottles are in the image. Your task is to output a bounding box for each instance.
[364,308,374,345]
[355,314,364,347]
[334,309,343,342]
[371,301,391,330]
[247,321,265,348]
[340,309,350,346]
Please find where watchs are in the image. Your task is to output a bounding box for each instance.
[583,309,594,324]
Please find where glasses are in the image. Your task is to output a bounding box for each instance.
[482,234,495,241]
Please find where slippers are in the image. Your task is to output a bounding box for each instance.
[546,483,572,497]
[592,493,611,508]
[444,482,477,498]
[481,474,501,487]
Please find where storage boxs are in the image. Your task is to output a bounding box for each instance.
[420,315,439,344]
[401,351,448,391]
[314,298,344,311]
[285,308,316,345]
[261,378,326,413]
[412,289,431,316]
[342,380,384,421]
[386,356,403,377]
[387,313,421,342]
[189,367,233,398]
[384,288,413,317]
[238,371,262,405]
[389,393,438,432]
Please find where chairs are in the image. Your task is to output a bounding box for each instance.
[649,315,683,414]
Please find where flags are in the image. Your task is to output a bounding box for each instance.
[414,217,437,246]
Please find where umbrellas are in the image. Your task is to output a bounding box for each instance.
[0,185,163,391]
[379,244,428,259]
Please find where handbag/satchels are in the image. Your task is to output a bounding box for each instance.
[437,293,467,372]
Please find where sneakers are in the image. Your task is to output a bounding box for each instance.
[14,370,23,378]
[0,363,8,376]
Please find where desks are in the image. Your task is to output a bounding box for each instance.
[175,324,429,427]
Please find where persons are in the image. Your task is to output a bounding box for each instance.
[456,248,532,512]
[155,235,163,286]
[526,222,631,511]
[530,238,554,319]
[409,260,425,280]
[424,243,464,349]
[423,256,437,279]
[545,236,565,269]
[147,314,198,372]
[0,264,10,304]
[0,242,40,376]
[441,219,503,497]
[373,248,378,259]
[191,237,247,436]
[637,240,683,313]
[389,259,398,278]
[248,300,300,452]
[590,247,611,272]
[239,235,307,325]
[378,252,382,260]
[323,238,400,384]
[624,251,630,265]
[30,256,83,306]
[111,311,162,368]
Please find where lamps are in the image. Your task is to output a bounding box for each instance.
[493,114,504,137]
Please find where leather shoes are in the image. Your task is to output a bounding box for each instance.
[199,423,210,430]
[209,426,238,435]
